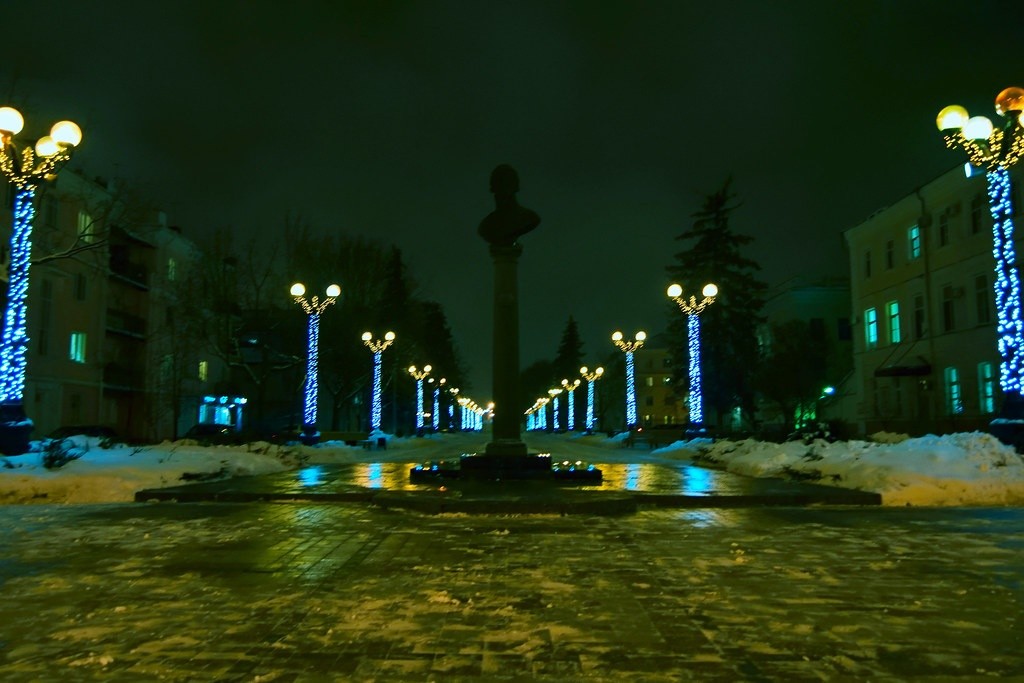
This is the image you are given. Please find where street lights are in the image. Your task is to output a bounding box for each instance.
[580,366,604,435]
[408,365,432,438]
[427,378,446,433]
[362,332,395,434]
[667,284,721,425]
[561,379,581,431]
[0,106,82,457]
[524,398,550,432]
[548,388,563,432]
[612,331,646,429]
[449,388,485,432]
[936,87,1024,456]
[290,283,341,446]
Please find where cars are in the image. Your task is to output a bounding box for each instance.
[281,423,304,432]
[46,425,114,440]
[173,424,236,446]
[439,428,455,433]
